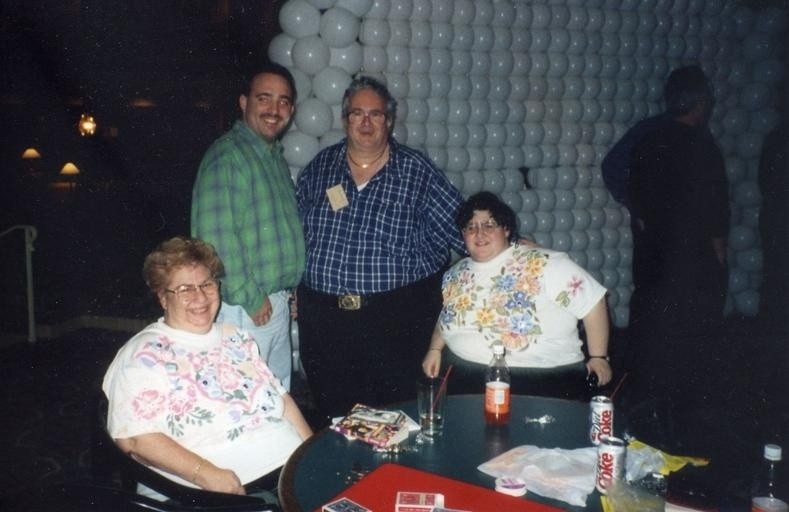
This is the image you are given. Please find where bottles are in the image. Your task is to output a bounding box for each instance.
[484,345,511,424]
[749,443,787,512]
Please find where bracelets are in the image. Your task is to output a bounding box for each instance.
[190,457,209,485]
[428,346,444,355]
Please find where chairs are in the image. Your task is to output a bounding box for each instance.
[89,364,271,512]
[584,351,620,403]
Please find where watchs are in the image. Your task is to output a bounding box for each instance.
[588,354,610,362]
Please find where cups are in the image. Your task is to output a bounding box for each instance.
[417,375,447,436]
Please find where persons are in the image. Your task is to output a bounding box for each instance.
[755,81,789,342]
[289,74,537,423]
[102,238,318,510]
[190,64,306,403]
[420,194,625,433]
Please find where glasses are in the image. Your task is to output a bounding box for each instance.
[164,277,222,306]
[344,108,389,128]
[461,220,501,237]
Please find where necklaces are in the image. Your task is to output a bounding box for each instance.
[344,142,389,168]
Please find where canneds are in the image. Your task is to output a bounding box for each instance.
[589,396,614,445]
[595,437,626,496]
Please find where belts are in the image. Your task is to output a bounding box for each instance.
[307,272,441,311]
